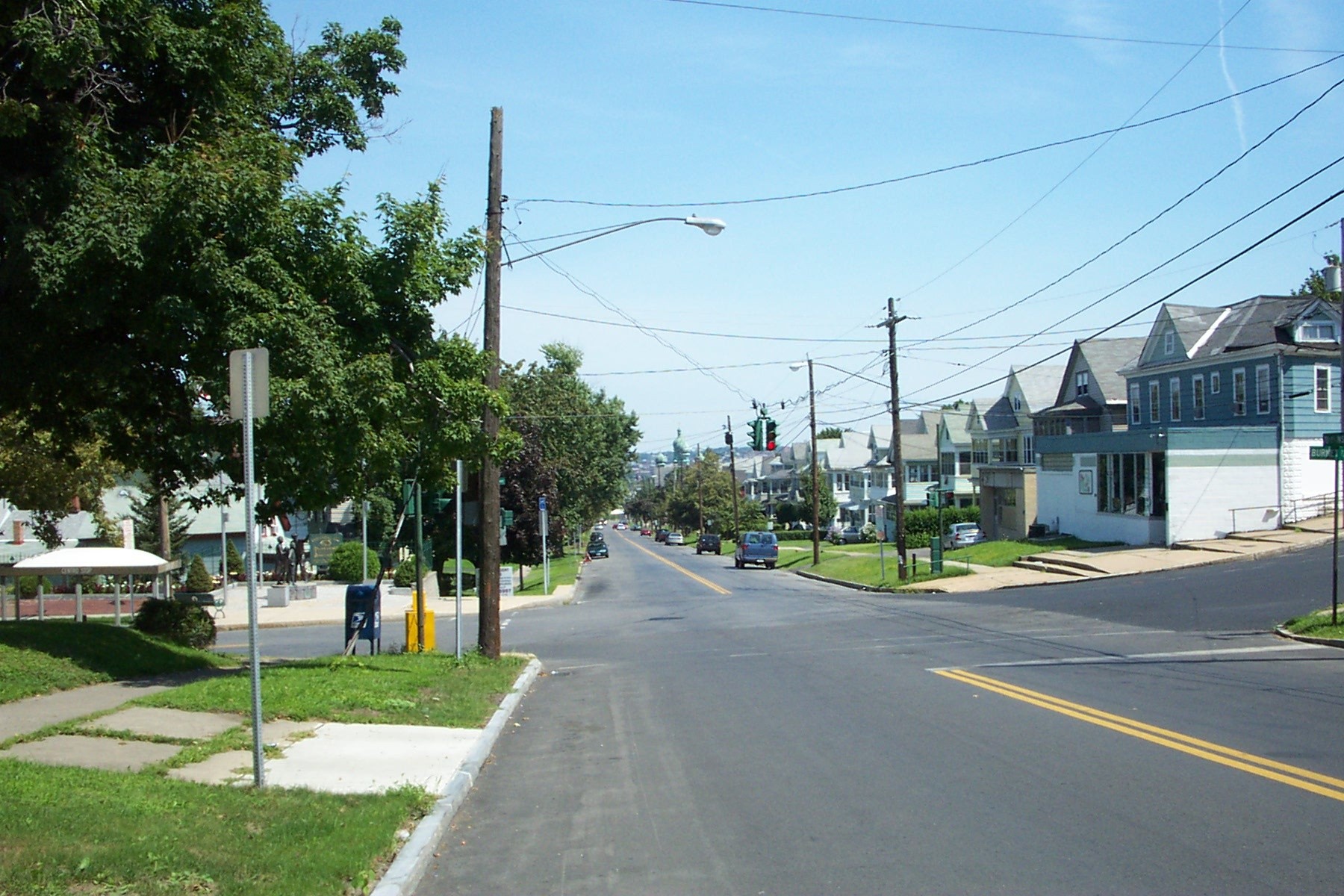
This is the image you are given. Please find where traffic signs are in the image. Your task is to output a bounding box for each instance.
[1310,445,1344,461]
[1323,432,1344,447]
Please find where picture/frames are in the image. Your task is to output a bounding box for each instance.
[1079,469,1093,494]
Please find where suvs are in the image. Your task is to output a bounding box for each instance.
[696,534,721,555]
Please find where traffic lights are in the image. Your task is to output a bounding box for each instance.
[747,420,763,451]
[766,420,779,451]
[427,488,453,514]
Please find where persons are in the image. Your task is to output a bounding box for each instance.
[290,533,310,581]
[288,542,296,585]
[276,536,289,585]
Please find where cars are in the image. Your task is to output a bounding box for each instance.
[631,525,651,537]
[836,525,860,545]
[655,529,683,545]
[590,532,603,541]
[587,541,609,559]
[943,522,987,550]
[593,523,603,529]
[617,523,625,530]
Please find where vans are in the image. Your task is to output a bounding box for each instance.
[733,531,778,570]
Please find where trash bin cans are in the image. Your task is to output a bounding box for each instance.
[345,585,381,656]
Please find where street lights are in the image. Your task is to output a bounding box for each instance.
[478,213,726,662]
[790,360,906,581]
